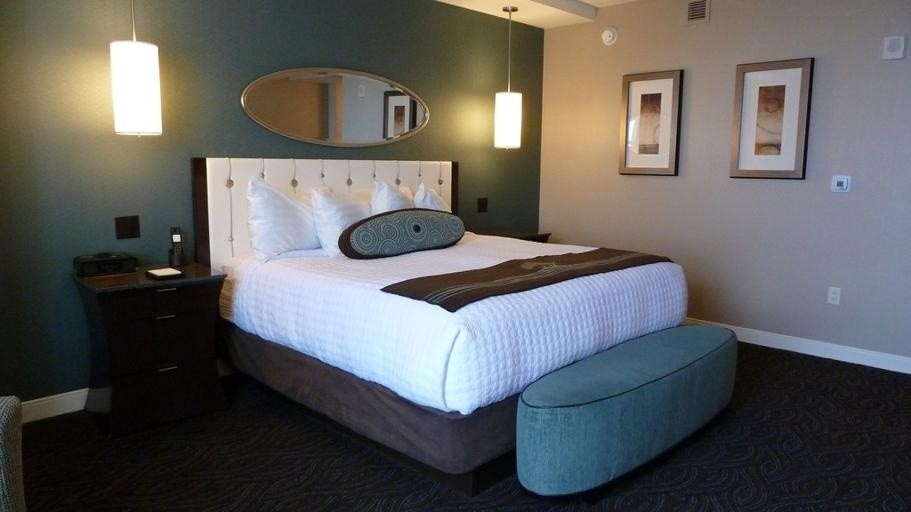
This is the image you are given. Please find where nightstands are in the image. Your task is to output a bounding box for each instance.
[470,230,552,243]
[79,263,231,445]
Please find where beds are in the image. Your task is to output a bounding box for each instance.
[190,157,688,498]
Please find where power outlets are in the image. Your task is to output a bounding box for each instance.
[831,175,851,194]
[827,287,841,305]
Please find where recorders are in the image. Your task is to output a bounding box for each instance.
[73,251,139,275]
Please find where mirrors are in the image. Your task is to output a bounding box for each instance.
[241,67,430,147]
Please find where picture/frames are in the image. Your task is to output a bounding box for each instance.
[729,57,814,179]
[619,70,682,175]
[383,92,416,140]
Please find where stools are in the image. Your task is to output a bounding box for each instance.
[515,324,738,497]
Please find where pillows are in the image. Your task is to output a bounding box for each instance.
[414,182,454,215]
[338,208,466,259]
[246,177,329,264]
[309,186,372,259]
[372,181,414,218]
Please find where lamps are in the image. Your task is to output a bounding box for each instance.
[494,6,522,150]
[109,2,163,136]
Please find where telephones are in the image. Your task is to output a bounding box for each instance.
[168,226,186,267]
